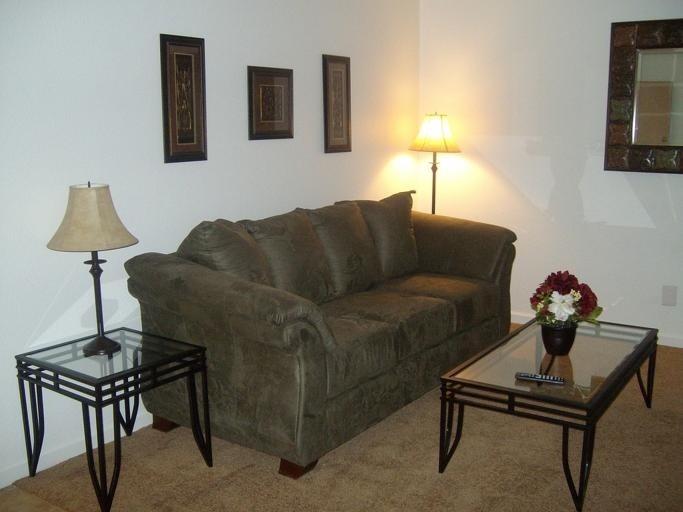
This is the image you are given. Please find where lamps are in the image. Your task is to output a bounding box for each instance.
[47,181,139,356]
[408,112,461,214]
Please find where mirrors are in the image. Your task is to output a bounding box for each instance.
[604,18,683,174]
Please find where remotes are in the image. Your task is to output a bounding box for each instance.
[514,372,565,385]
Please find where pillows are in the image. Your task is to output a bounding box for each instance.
[322,54,351,153]
[247,65,294,140]
[177,190,416,305]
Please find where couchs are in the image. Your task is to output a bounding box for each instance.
[124,190,517,479]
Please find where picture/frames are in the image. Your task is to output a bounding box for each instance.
[159,34,207,163]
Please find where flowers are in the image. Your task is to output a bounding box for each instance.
[529,270,603,326]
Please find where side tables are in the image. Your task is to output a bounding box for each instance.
[15,327,213,512]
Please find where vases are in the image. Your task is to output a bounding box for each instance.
[540,323,577,355]
